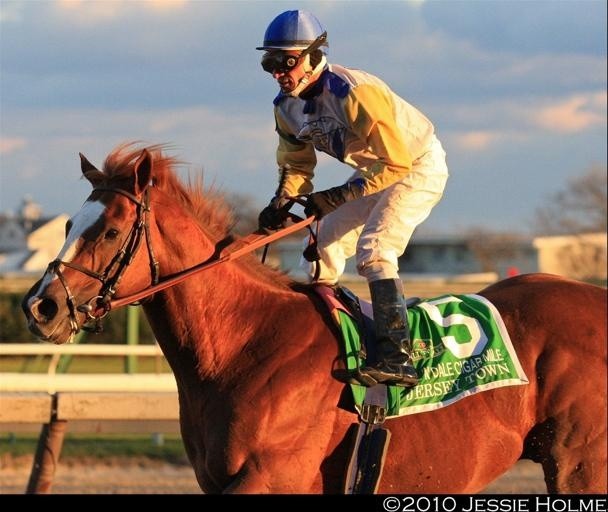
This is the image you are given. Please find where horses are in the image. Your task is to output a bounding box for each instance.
[21,138,607,498]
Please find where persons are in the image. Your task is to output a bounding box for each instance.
[255,8,451,388]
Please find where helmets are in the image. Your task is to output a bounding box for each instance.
[256,9,329,54]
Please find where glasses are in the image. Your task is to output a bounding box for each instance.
[261,33,327,72]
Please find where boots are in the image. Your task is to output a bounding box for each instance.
[347,278,419,387]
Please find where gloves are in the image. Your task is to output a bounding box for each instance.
[304,186,346,222]
[258,196,292,235]
